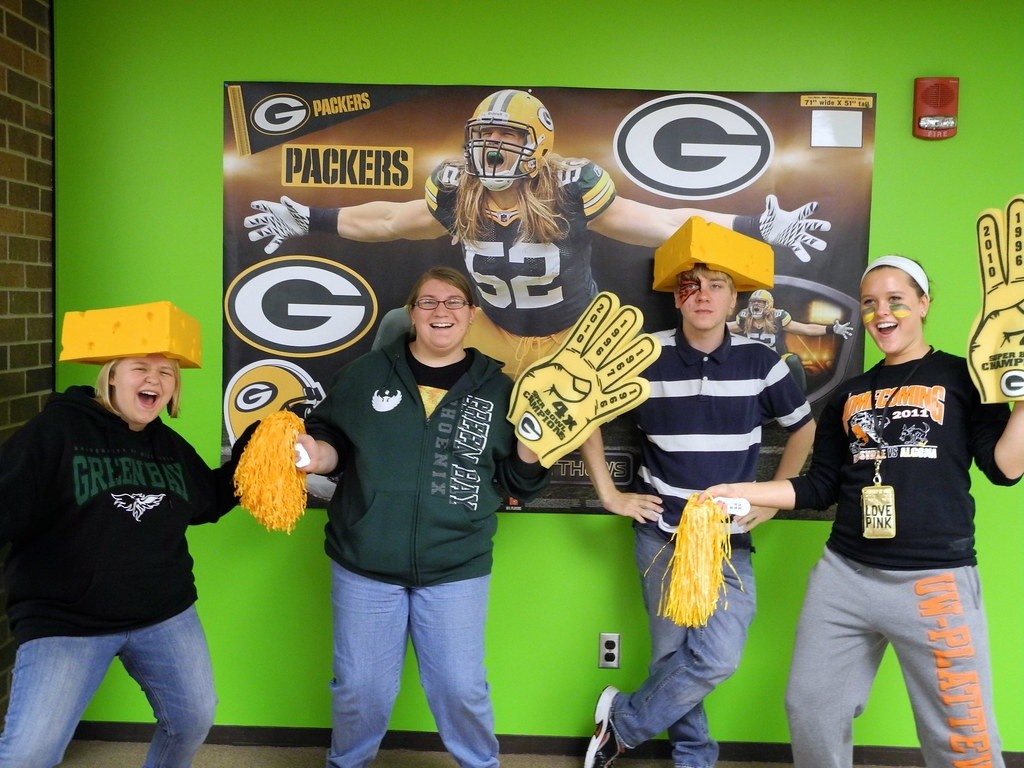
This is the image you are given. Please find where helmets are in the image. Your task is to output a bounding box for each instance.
[469,89,554,178]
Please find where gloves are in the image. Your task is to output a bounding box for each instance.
[967,197,1024,406]
[732,194,832,263]
[244,196,338,255]
[506,292,662,467]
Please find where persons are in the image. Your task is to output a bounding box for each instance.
[726,289,854,391]
[244,87,832,371]
[290,266,572,768]
[696,257,1024,768]
[581,249,817,768]
[1,333,293,768]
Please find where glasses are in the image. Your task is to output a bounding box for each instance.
[415,297,468,310]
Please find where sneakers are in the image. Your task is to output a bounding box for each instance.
[585,686,626,768]
